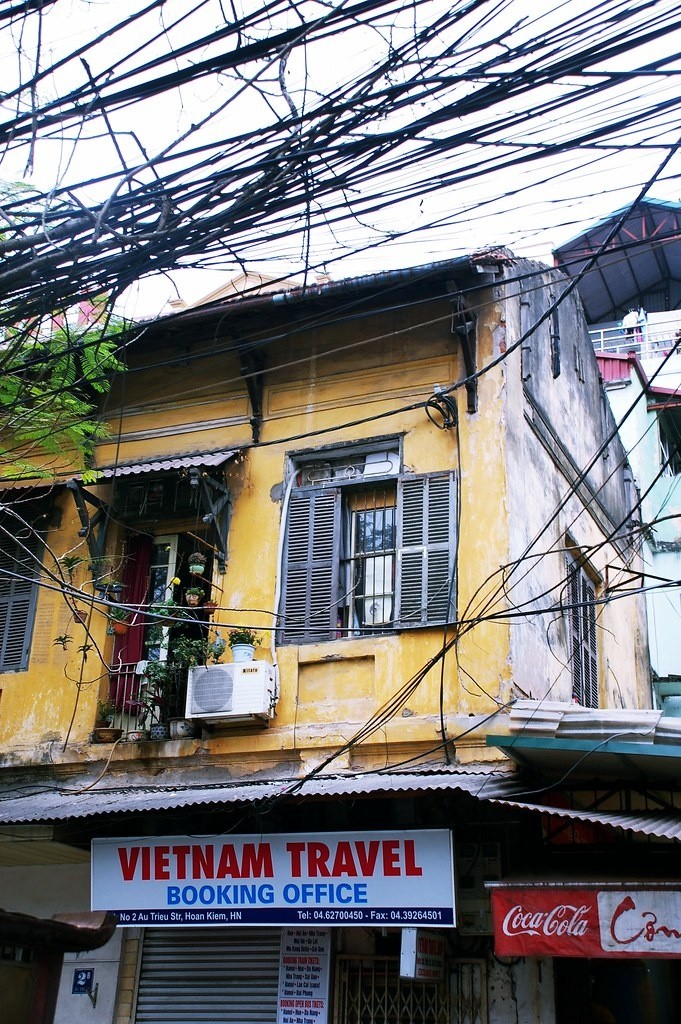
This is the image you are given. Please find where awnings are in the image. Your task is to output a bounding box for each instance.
[489,796,681,840]
[0,765,524,824]
[0,445,240,491]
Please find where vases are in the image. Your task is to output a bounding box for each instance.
[126,729,148,742]
[230,644,257,663]
[186,595,201,606]
[74,611,88,623]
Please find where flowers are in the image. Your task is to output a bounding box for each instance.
[228,627,263,647]
[185,587,205,597]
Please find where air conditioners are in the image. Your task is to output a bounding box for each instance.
[186,659,274,724]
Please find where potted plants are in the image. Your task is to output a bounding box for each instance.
[188,552,207,575]
[204,600,218,614]
[125,685,170,741]
[93,697,124,743]
[111,608,133,634]
[146,660,196,740]
